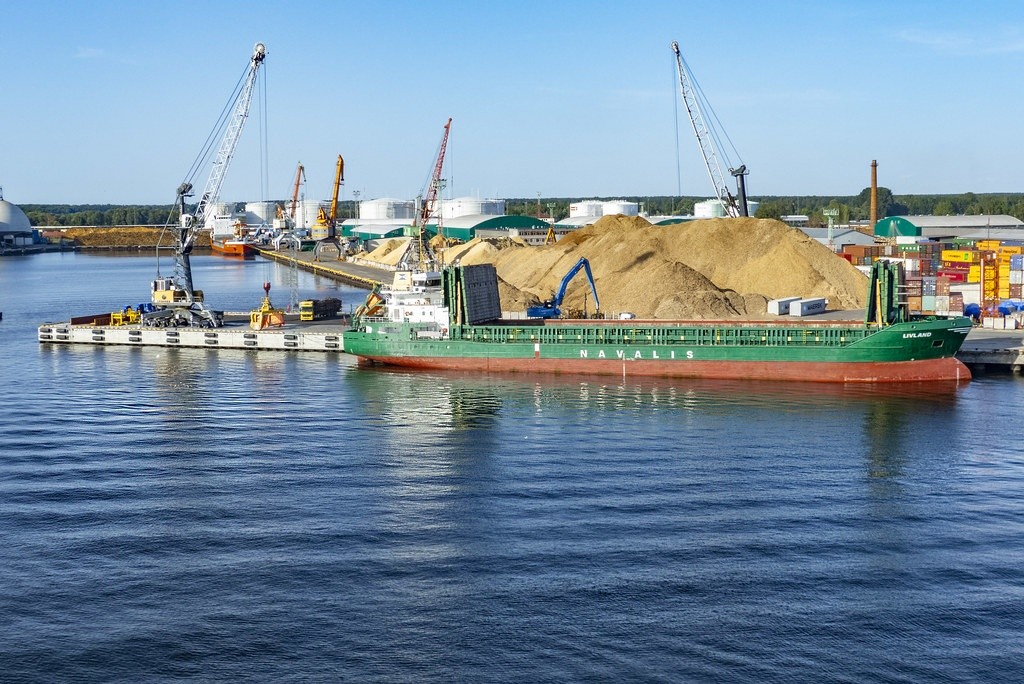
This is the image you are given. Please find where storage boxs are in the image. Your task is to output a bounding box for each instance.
[832,238,1024,331]
[767,297,826,317]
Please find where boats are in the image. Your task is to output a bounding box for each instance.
[210,218,256,253]
[344,259,973,383]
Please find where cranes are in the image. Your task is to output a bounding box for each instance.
[308,154,344,262]
[272,163,307,252]
[398,117,452,284]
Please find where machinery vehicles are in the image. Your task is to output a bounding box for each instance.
[670,40,752,217]
[526,257,603,319]
[135,40,270,326]
